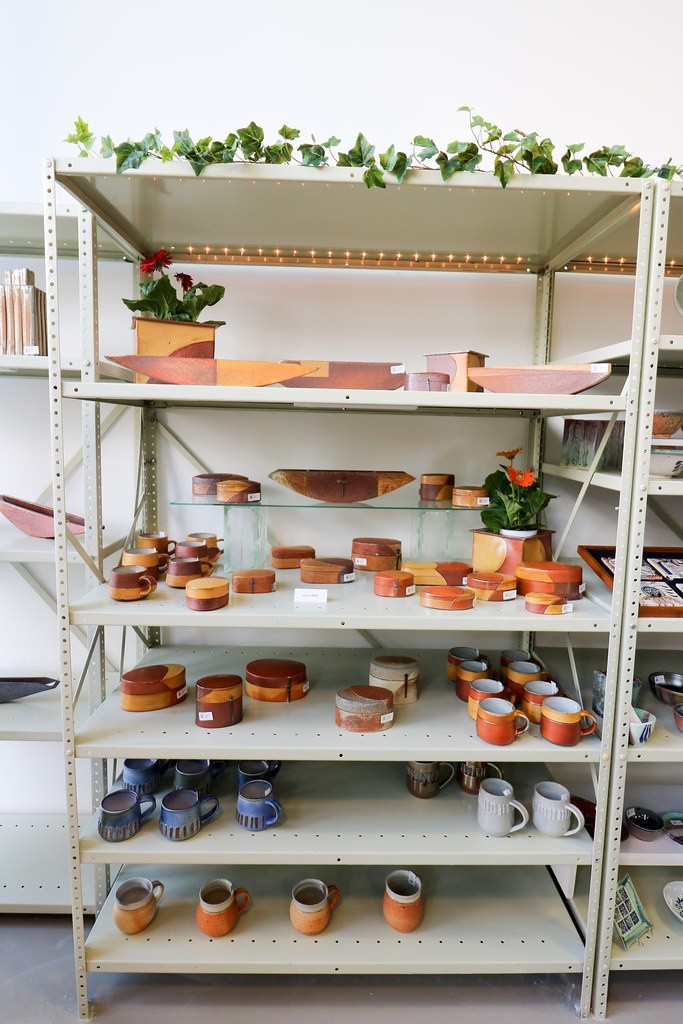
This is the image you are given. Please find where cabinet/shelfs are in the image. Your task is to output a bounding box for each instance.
[0,154,682,1021]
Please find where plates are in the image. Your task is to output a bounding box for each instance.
[663,881,683,924]
[661,812,683,846]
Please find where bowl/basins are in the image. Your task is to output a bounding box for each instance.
[652,409,683,439]
[626,807,664,841]
[649,448,683,479]
[629,671,683,747]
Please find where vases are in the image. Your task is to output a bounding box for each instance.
[469,528,556,595]
[423,350,488,392]
[130,315,220,384]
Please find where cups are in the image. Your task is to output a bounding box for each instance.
[460,762,504,795]
[290,878,340,936]
[166,532,224,588]
[447,647,569,724]
[382,870,423,932]
[197,879,249,937]
[109,565,157,600]
[477,697,529,745]
[533,781,585,837]
[97,758,282,841]
[113,877,165,935]
[406,762,455,799]
[541,696,597,747]
[477,779,529,837]
[138,531,177,555]
[122,548,171,581]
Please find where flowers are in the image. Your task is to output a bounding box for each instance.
[480,446,563,531]
[121,249,226,327]
